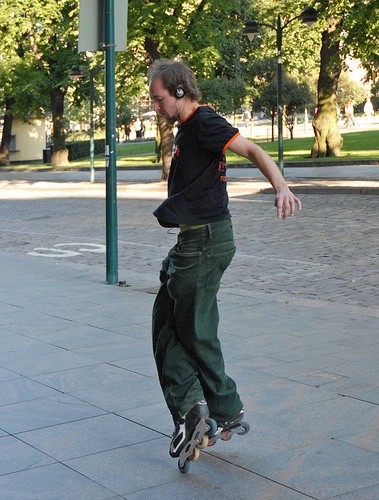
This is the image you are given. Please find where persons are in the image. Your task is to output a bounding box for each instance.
[147,58,301,474]
[124,118,146,141]
[313,100,355,128]
[364,97,374,123]
[242,107,251,128]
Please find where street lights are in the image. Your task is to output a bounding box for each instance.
[241,4,320,177]
[71,64,105,183]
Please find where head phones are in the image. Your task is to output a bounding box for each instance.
[175,87,185,98]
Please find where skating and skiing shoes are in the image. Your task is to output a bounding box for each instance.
[207,406,250,447]
[168,400,217,473]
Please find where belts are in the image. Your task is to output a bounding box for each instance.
[180,224,207,233]
